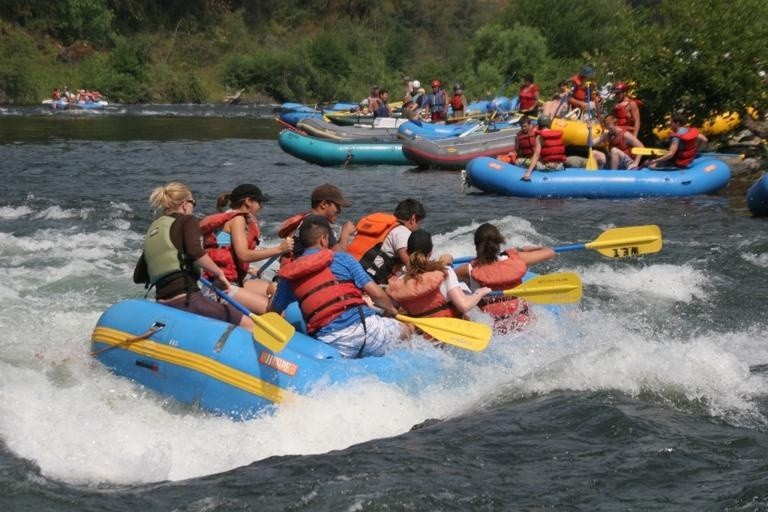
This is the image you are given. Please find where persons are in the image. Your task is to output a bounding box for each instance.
[133,181,256,331]
[198,183,295,319]
[276,183,356,268]
[368,89,392,118]
[644,118,708,170]
[612,83,640,137]
[368,84,379,102]
[569,94,602,121]
[52,86,103,104]
[439,223,555,335]
[513,114,538,159]
[560,67,593,120]
[513,73,540,118]
[425,80,449,122]
[514,114,566,181]
[268,215,415,359]
[585,114,645,172]
[385,230,493,348]
[402,93,429,118]
[558,80,572,98]
[448,84,466,118]
[404,76,413,95]
[410,80,425,112]
[349,198,426,285]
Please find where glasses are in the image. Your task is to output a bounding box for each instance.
[186,197,197,207]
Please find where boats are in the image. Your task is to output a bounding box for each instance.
[278,97,545,140]
[284,112,329,125]
[745,172,767,217]
[299,115,401,145]
[278,128,406,165]
[403,128,518,165]
[90,257,579,421]
[41,99,107,110]
[548,107,759,152]
[465,155,731,197]
[41,98,109,110]
[89,256,605,424]
[399,131,521,166]
[464,155,732,197]
[281,130,401,163]
[400,119,512,136]
[283,97,513,116]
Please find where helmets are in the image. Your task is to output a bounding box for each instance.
[537,116,551,127]
[609,82,630,92]
[453,84,462,90]
[430,81,441,87]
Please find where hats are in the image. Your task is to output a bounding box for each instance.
[229,183,270,204]
[311,182,352,207]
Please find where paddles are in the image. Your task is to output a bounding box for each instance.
[631,146,746,161]
[462,271,583,305]
[373,306,492,354]
[452,224,664,263]
[585,81,599,168]
[198,276,296,355]
